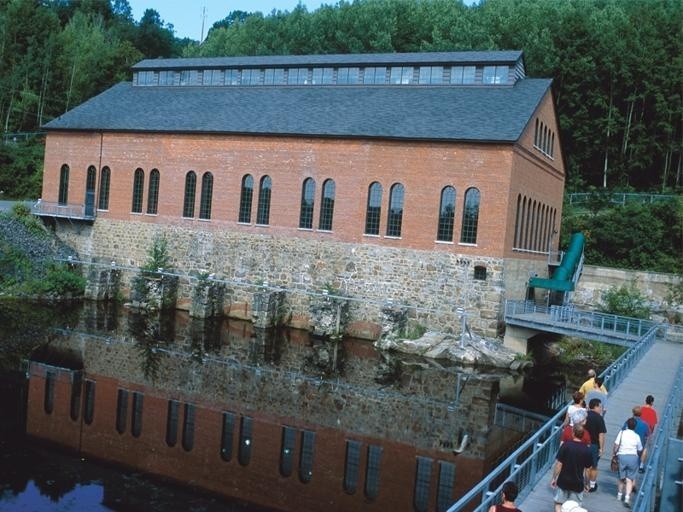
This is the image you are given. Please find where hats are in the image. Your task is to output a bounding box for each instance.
[571,408,588,424]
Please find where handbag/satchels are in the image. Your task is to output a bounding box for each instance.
[609,456,618,472]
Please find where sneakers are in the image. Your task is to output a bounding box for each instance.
[590,467,645,509]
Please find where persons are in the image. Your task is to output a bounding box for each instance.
[622,407,650,492]
[613,419,644,507]
[551,369,608,512]
[639,394,657,473]
[487,482,522,512]
